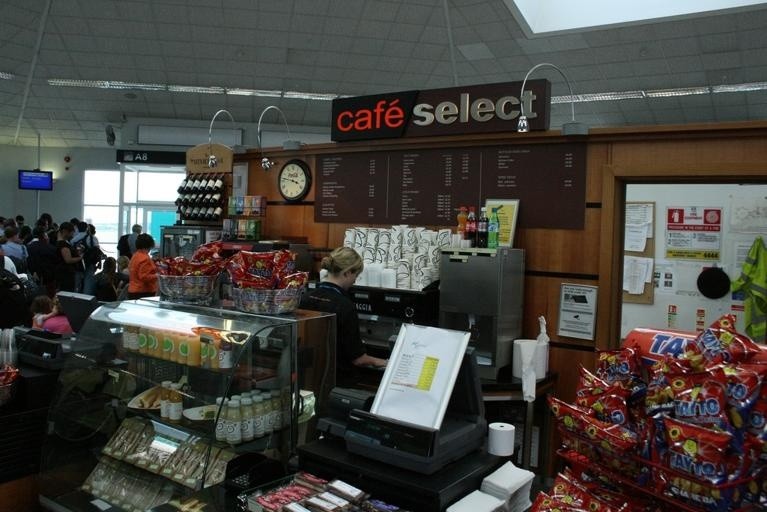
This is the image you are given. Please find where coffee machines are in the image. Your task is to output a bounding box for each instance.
[345,285,440,360]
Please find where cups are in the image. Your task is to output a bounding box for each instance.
[343,224,471,292]
[0,328,19,363]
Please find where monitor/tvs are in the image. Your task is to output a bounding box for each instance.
[18,170,53,190]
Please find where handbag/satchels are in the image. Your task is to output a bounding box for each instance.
[19,271,40,302]
[26,237,61,272]
[83,235,106,271]
[9,255,26,274]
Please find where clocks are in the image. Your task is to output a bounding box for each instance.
[277,157,311,201]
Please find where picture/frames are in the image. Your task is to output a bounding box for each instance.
[557,282,598,341]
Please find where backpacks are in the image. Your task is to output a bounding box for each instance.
[58,244,84,271]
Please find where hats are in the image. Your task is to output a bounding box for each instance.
[0,269,33,328]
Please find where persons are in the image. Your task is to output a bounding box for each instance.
[113,279,130,296]
[299,246,387,386]
[118,256,131,275]
[0,213,117,336]
[128,233,159,300]
[117,224,142,260]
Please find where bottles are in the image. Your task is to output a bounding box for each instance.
[160,381,183,423]
[456,206,500,248]
[121,318,235,373]
[214,389,283,445]
[175,171,228,221]
[281,386,290,431]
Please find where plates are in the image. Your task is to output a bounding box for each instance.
[126,385,161,409]
[182,405,217,421]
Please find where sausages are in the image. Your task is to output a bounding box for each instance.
[257,472,327,510]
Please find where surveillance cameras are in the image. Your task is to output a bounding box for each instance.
[105,124,116,146]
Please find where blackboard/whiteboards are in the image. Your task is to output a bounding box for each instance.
[314,143,586,230]
[609,175,767,352]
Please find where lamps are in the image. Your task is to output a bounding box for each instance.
[516,62,588,136]
[256,105,302,171]
[206,109,245,167]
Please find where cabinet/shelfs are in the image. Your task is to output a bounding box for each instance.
[181,172,231,227]
[555,327,766,511]
[36,297,334,511]
